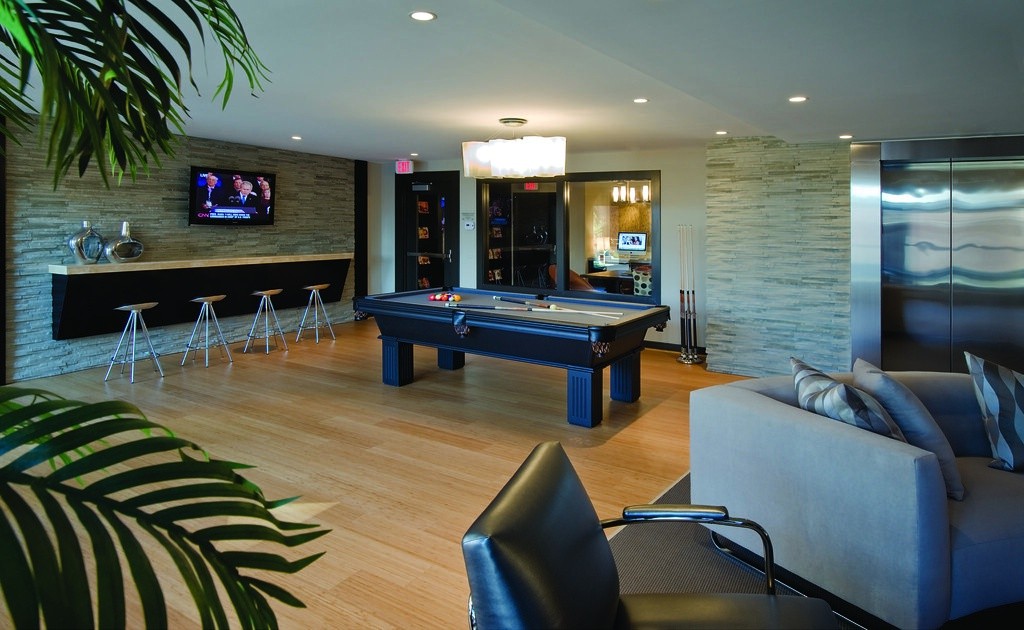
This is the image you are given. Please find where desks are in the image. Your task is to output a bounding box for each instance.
[579,270,634,295]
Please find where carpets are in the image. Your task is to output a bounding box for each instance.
[606,466,1022,630]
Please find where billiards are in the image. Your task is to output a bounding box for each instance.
[549,304,557,310]
[427,291,461,301]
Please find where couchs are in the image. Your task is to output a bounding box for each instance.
[680,369,1023,629]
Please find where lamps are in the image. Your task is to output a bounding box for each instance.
[459,118,567,180]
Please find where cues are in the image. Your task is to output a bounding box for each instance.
[444,303,623,315]
[689,224,701,363]
[681,225,694,364]
[677,224,682,361]
[681,225,688,363]
[493,296,618,319]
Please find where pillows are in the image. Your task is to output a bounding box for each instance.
[962,350,1024,478]
[787,354,907,446]
[850,359,965,502]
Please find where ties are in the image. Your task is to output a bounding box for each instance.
[242,195,246,204]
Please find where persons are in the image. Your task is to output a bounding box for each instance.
[622,235,642,245]
[196,172,272,222]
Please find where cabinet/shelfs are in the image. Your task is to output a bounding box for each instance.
[483,182,515,287]
[411,181,447,290]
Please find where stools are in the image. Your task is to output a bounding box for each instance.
[513,263,529,290]
[243,289,288,357]
[104,302,166,385]
[295,285,337,345]
[491,266,503,287]
[532,262,548,289]
[181,294,233,368]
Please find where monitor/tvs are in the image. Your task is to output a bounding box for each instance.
[617,231,647,256]
[189,165,277,227]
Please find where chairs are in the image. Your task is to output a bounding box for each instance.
[458,440,835,630]
[549,264,595,291]
[628,259,651,273]
[631,265,653,296]
